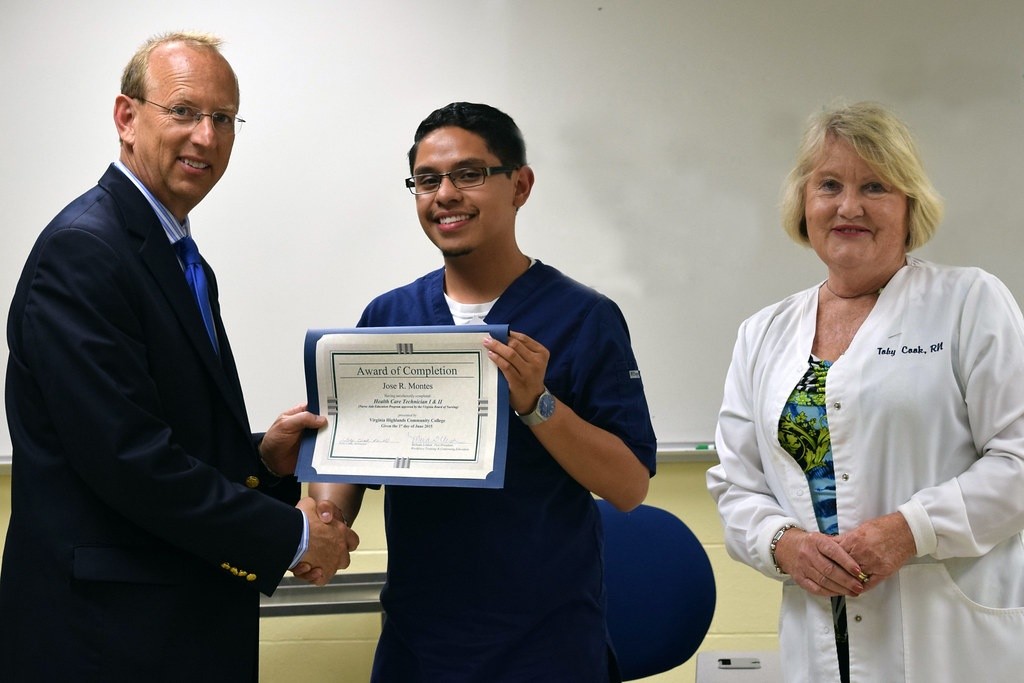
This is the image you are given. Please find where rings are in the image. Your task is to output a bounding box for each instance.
[856,573,869,584]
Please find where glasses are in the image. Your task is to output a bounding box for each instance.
[405,166,517,196]
[135,97,246,136]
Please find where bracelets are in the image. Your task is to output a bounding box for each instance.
[770,524,806,574]
[340,509,348,526]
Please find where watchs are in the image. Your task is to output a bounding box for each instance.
[515,385,558,425]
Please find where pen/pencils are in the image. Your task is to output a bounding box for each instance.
[696,444,717,451]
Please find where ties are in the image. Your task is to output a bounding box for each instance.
[172,237,219,358]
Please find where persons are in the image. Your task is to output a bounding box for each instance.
[1,30,361,683]
[293,103,657,683]
[703,103,1023,683]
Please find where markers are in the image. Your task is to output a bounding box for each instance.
[0,0,1024,465]
